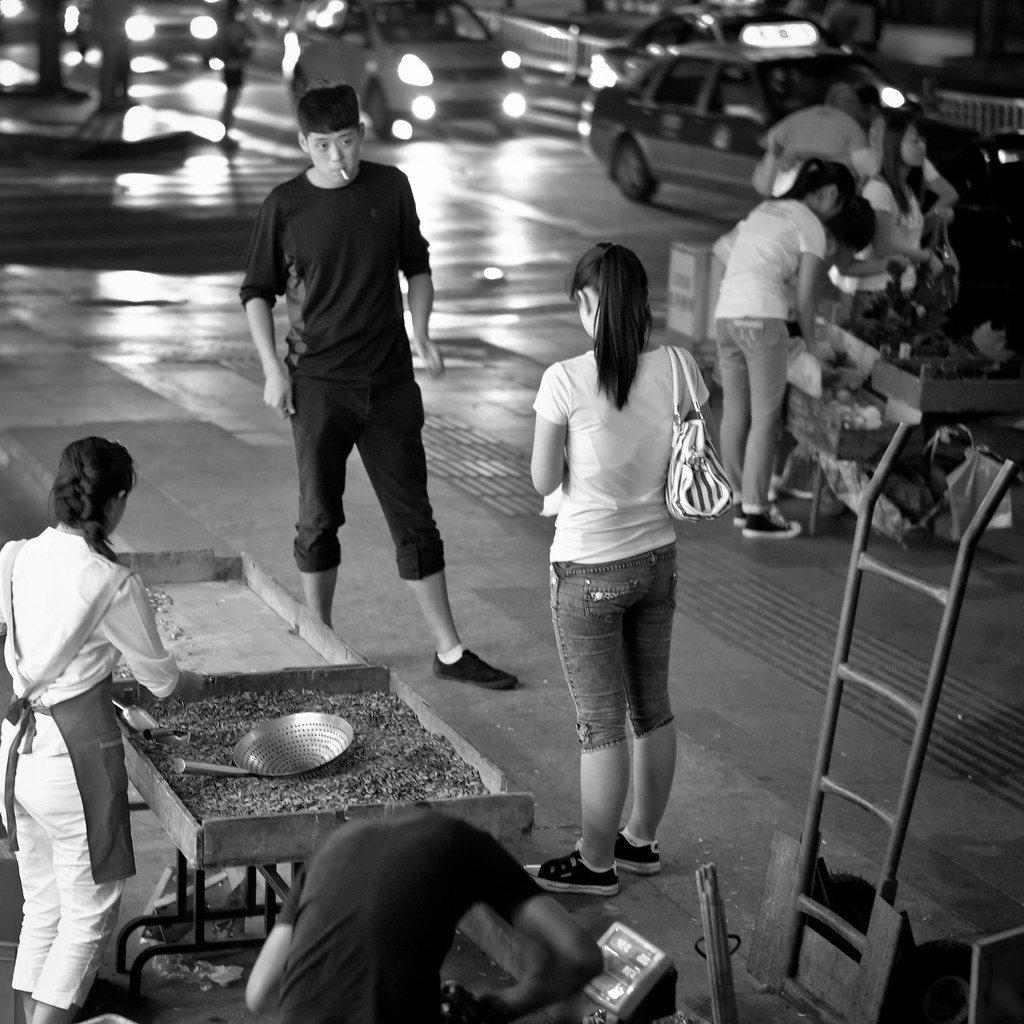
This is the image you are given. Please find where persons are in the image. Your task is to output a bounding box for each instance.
[246,801,604,1024]
[523,242,718,896]
[240,84,517,693]
[0,437,201,1024]
[217,1,253,133]
[704,82,958,542]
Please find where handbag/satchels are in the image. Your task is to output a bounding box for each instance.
[920,218,959,311]
[665,346,734,522]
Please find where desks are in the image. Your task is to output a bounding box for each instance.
[102,548,537,1024]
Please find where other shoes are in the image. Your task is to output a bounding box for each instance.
[434,649,518,690]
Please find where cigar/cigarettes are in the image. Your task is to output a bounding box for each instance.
[338,168,349,181]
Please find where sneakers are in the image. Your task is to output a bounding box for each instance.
[733,497,802,538]
[524,851,619,896]
[574,828,661,875]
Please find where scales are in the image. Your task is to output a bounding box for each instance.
[581,920,679,1024]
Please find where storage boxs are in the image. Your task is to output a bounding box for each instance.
[827,303,1024,414]
[663,239,724,341]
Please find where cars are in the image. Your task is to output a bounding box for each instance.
[280,0,549,140]
[1,0,257,108]
[581,1,1023,373]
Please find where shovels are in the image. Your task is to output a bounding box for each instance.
[143,726,199,746]
[112,697,160,732]
